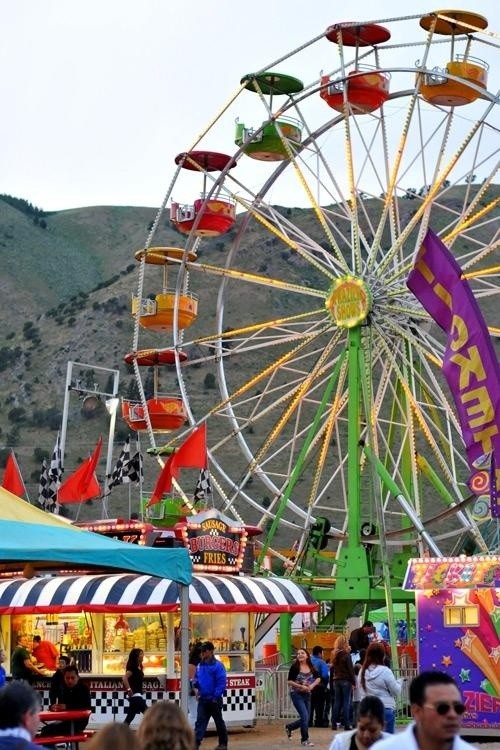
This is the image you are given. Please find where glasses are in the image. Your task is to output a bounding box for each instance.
[422,702,466,716]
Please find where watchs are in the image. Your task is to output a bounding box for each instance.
[126,687,131,691]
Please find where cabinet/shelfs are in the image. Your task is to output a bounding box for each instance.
[1,613,255,674]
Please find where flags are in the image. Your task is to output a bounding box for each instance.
[0,451,26,498]
[34,434,145,513]
[146,421,210,509]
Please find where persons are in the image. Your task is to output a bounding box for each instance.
[281,620,480,750]
[187,640,230,750]
[85,720,140,750]
[138,700,196,750]
[0,633,92,750]
[120,647,150,727]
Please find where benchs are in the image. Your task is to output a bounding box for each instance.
[32,730,95,750]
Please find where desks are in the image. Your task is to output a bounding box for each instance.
[37,709,92,733]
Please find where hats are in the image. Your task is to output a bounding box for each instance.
[198,641,214,650]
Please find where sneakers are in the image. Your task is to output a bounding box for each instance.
[285,724,292,740]
[300,738,314,747]
[215,746,227,750]
